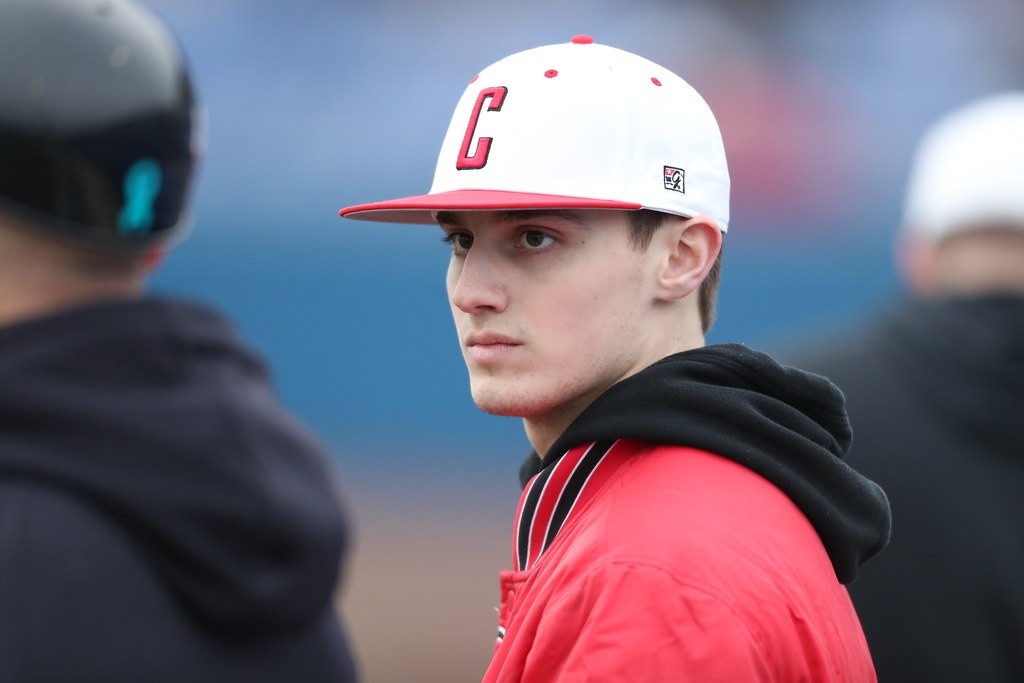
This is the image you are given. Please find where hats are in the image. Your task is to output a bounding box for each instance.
[339,34,730,234]
[902,92,1024,238]
[0,0,196,240]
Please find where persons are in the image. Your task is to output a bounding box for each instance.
[337,34,893,683]
[0,0,358,683]
[781,92,1024,683]
